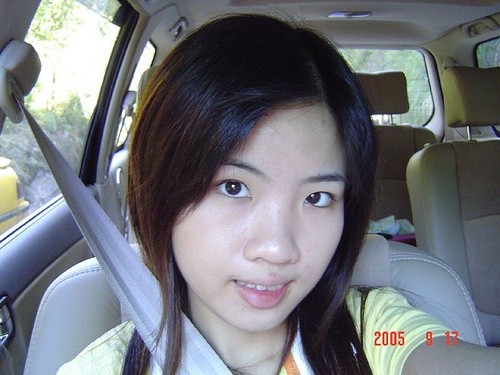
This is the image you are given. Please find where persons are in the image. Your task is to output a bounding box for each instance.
[52,11,500,375]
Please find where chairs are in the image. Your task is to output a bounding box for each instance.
[353,70,439,242]
[21,234,487,375]
[405,66,499,346]
